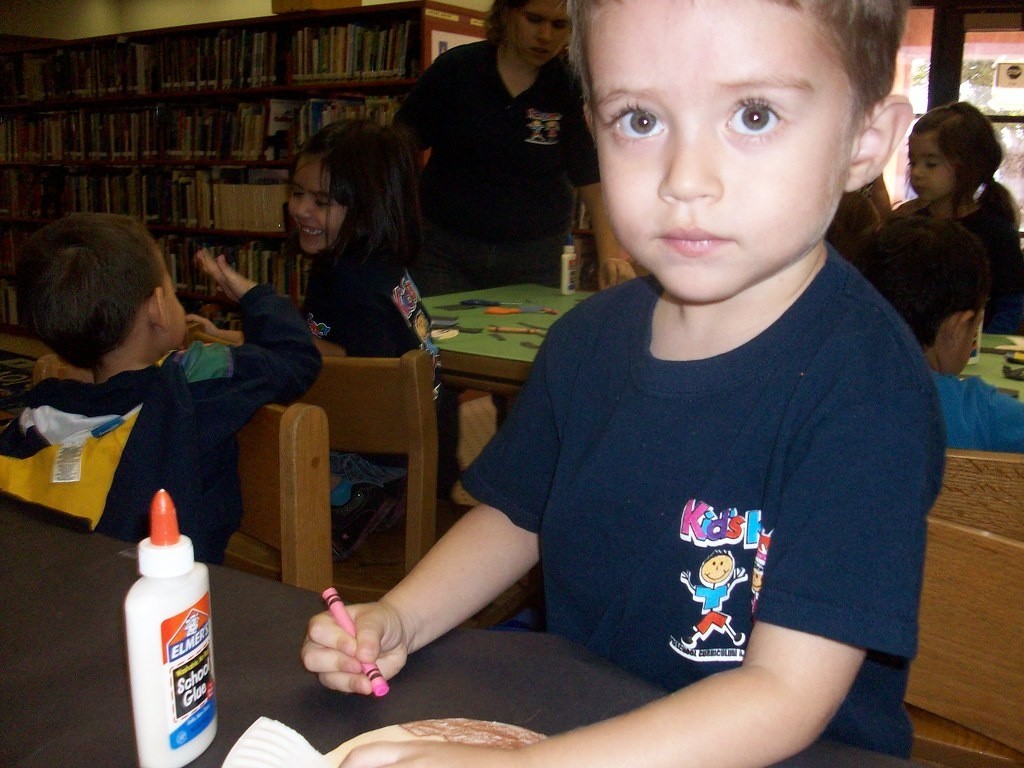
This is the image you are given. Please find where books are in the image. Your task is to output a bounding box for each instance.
[0,18,409,331]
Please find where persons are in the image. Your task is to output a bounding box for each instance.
[1,213,320,566]
[303,0,944,768]
[849,218,1024,452]
[828,101,1023,333]
[185,119,437,563]
[392,0,640,296]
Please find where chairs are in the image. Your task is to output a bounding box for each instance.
[901,449,1024,768]
[31,322,526,631]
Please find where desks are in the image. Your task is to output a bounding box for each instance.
[419,283,1024,429]
[0,505,927,768]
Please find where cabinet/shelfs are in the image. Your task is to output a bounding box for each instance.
[0,0,433,328]
